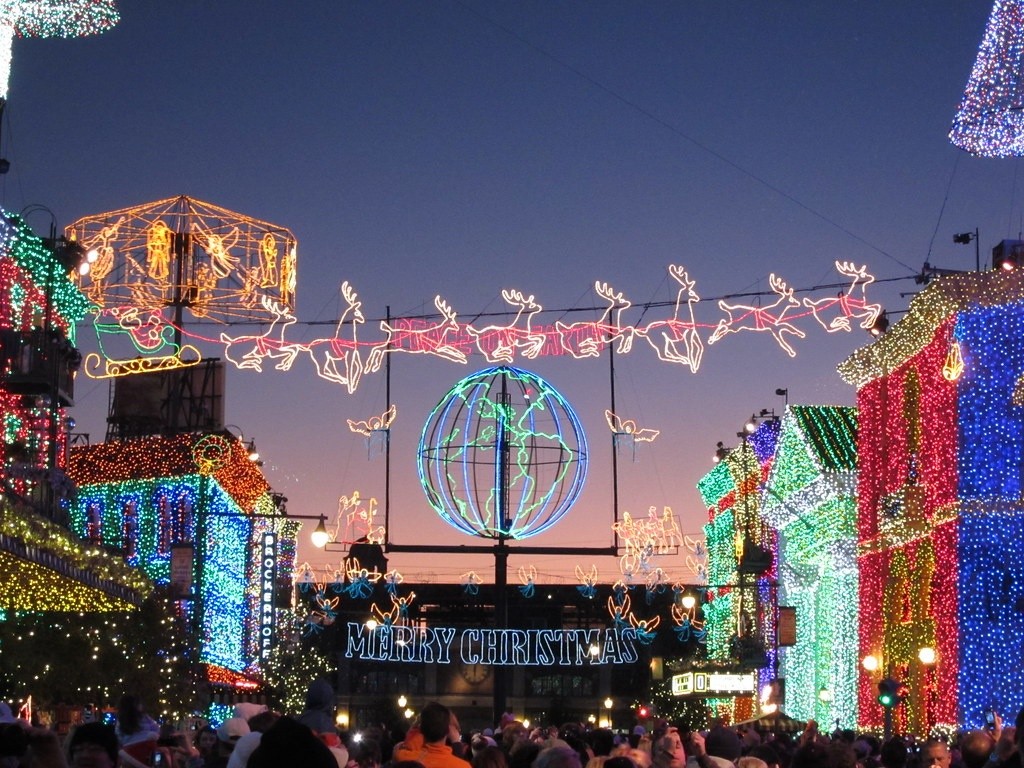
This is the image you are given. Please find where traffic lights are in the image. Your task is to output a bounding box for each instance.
[877,680,898,708]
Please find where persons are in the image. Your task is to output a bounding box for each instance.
[0,696,1024,768]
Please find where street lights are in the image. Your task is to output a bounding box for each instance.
[590,639,658,717]
[862,654,892,735]
[682,583,780,714]
[918,646,938,737]
[604,697,613,729]
[189,510,330,704]
